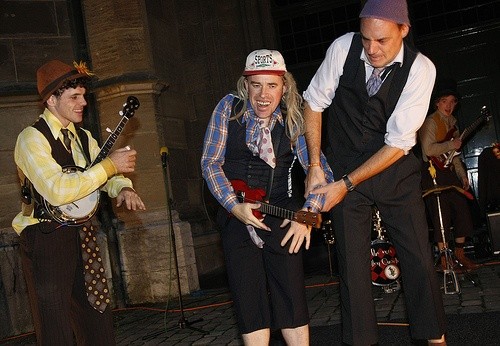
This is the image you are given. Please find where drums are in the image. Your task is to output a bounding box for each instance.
[369,240,401,285]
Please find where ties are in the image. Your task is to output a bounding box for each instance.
[365,67,384,96]
[257,119,276,168]
[61,129,110,314]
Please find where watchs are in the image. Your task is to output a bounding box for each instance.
[342,174,357,191]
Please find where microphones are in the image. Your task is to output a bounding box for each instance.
[160,146,168,168]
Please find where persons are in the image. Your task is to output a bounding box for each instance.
[302,1,448,346]
[11,59,146,346]
[419,89,471,271]
[200,49,335,346]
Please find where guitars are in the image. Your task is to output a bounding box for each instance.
[40,96,140,227]
[427,106,491,172]
[210,180,322,228]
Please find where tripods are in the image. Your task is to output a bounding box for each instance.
[432,178,478,294]
[142,156,209,340]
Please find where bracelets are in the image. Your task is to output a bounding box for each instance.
[307,163,322,167]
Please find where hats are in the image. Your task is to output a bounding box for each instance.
[360,0,410,27]
[37,60,96,104]
[242,49,287,75]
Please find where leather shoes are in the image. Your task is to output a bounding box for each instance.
[461,244,475,249]
[440,247,455,256]
